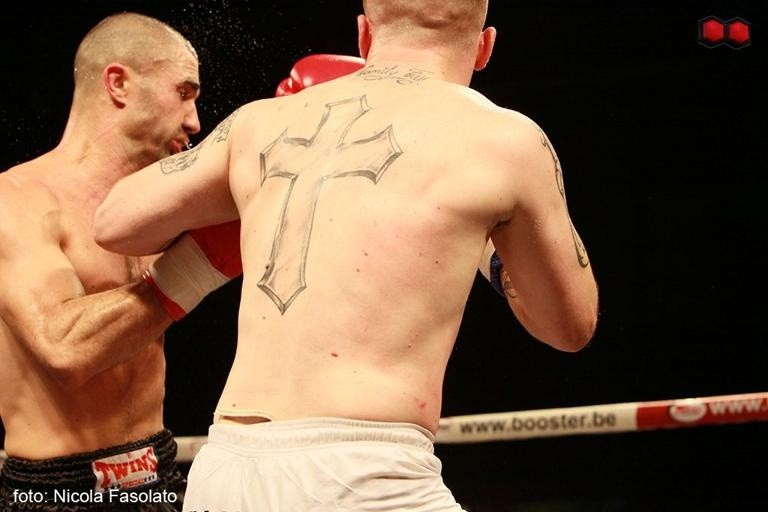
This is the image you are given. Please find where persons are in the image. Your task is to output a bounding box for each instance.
[92,0,600,511]
[1,12,367,511]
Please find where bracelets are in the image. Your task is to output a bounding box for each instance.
[489,249,506,299]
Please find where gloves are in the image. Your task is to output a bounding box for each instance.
[276,53,366,96]
[141,221,241,320]
